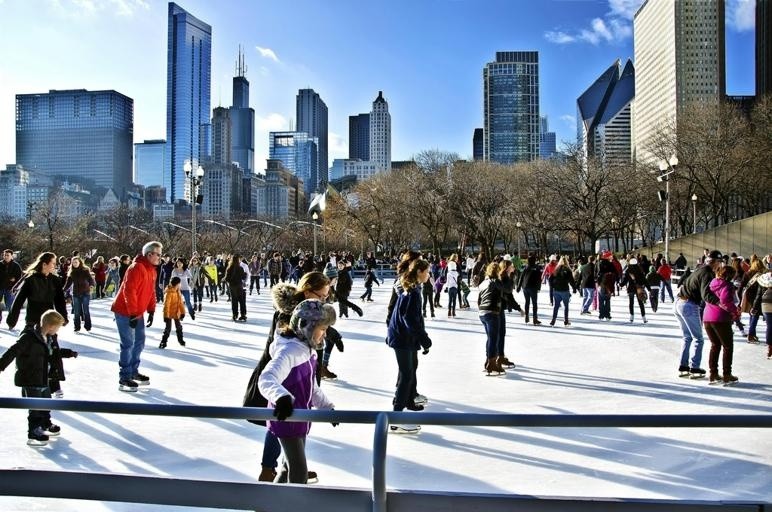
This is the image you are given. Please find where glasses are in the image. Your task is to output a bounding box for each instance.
[192,260,197,262]
[152,254,161,257]
[3,255,10,257]
[311,291,329,301]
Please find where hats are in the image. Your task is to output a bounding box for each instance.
[513,249,518,254]
[210,256,214,261]
[679,253,682,255]
[634,245,639,249]
[323,263,338,277]
[439,276,447,283]
[450,254,457,260]
[630,259,637,264]
[289,298,336,330]
[73,251,79,256]
[601,248,614,258]
[551,255,556,260]
[731,253,737,257]
[504,254,510,260]
[708,250,723,259]
[685,267,690,271]
[273,252,280,257]
[448,261,456,270]
[661,259,666,264]
[338,261,344,264]
[649,266,656,272]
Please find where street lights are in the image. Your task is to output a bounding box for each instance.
[182,163,205,255]
[515,220,522,259]
[309,209,320,257]
[689,192,698,234]
[654,156,679,268]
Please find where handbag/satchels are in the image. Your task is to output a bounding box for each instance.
[637,288,648,301]
[471,275,480,287]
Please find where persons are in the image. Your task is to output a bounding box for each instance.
[243,246,524,484]
[517,249,771,386]
[1,240,264,447]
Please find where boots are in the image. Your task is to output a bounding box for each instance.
[210,297,213,302]
[431,310,435,316]
[199,304,202,311]
[525,314,529,322]
[257,290,260,294]
[533,317,541,323]
[215,296,218,302]
[485,357,505,372]
[193,304,197,311]
[767,345,771,357]
[249,290,252,295]
[423,310,426,317]
[320,366,337,378]
[460,304,464,307]
[448,310,451,316]
[452,310,456,316]
[739,325,744,332]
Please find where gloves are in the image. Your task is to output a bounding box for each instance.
[423,349,429,354]
[146,313,153,327]
[71,351,77,358]
[129,315,137,328]
[355,305,363,316]
[272,396,293,420]
[336,340,344,352]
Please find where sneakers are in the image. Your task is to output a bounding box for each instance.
[709,374,721,381]
[630,315,634,320]
[464,304,466,307]
[367,298,373,301]
[191,312,195,320]
[28,428,49,441]
[233,313,238,319]
[119,379,138,387]
[581,311,591,314]
[394,399,424,411]
[420,395,427,400]
[390,424,416,430]
[239,316,247,320]
[331,408,339,427]
[56,390,63,395]
[178,337,185,345]
[679,365,690,371]
[258,466,277,482]
[360,296,364,300]
[564,321,571,325]
[691,368,706,374]
[466,304,470,307]
[748,335,758,341]
[159,338,167,346]
[642,316,648,321]
[285,466,317,479]
[134,373,149,380]
[496,356,513,365]
[414,396,423,403]
[550,320,555,325]
[723,375,738,382]
[42,423,60,432]
